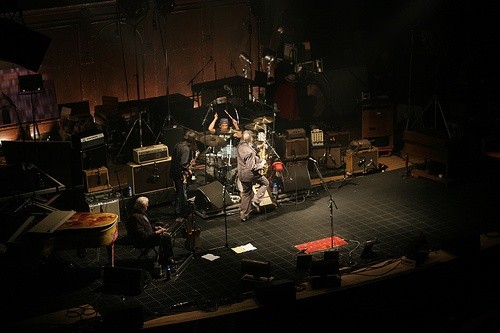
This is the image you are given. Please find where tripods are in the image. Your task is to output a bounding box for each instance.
[175,204,214,274]
[199,195,238,256]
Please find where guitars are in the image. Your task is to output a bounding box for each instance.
[257,125,268,175]
[182,150,200,184]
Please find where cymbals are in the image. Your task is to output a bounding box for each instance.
[175,125,199,139]
[197,135,225,147]
[253,116,274,123]
[246,123,265,130]
[219,130,239,134]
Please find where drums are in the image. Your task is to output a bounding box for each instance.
[226,168,242,192]
[218,135,242,159]
[206,154,228,178]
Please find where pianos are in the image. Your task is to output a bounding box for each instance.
[6,209,119,268]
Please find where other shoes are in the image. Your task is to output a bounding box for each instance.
[157,257,174,265]
[252,201,260,212]
[241,216,249,222]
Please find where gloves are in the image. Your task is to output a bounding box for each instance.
[255,163,266,171]
[257,143,268,150]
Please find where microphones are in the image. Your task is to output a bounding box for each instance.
[152,177,158,184]
[222,185,226,195]
[357,159,365,166]
[308,157,317,163]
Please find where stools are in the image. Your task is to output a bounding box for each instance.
[138,245,158,261]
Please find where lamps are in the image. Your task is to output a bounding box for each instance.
[238,26,323,73]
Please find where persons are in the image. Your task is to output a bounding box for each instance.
[127,196,178,265]
[237,130,269,222]
[208,113,242,138]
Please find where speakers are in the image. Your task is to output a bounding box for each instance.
[102,266,143,295]
[95,302,145,330]
[281,165,312,193]
[193,180,233,214]
[128,157,173,194]
[362,111,393,152]
[407,231,428,266]
[82,165,109,193]
[308,250,341,274]
[253,278,296,307]
[310,147,341,169]
[346,148,380,173]
[281,137,309,159]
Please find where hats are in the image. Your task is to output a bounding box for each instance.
[242,130,252,140]
[220,119,228,125]
